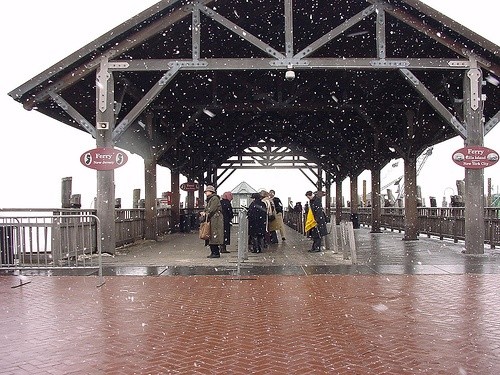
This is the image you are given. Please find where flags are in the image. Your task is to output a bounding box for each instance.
[305,208,317,232]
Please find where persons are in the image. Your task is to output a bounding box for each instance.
[305,191,327,253]
[290,201,308,214]
[219,191,233,253]
[200,185,224,258]
[247,190,286,253]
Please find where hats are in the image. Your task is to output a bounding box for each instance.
[204,185,215,193]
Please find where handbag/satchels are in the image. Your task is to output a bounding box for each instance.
[199,213,210,240]
[269,212,283,231]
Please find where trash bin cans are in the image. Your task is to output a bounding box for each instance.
[351,212,359,228]
[181,214,191,232]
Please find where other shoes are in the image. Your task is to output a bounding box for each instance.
[307,247,314,252]
[221,251,230,253]
[282,237,286,241]
[310,248,320,253]
[207,253,220,258]
